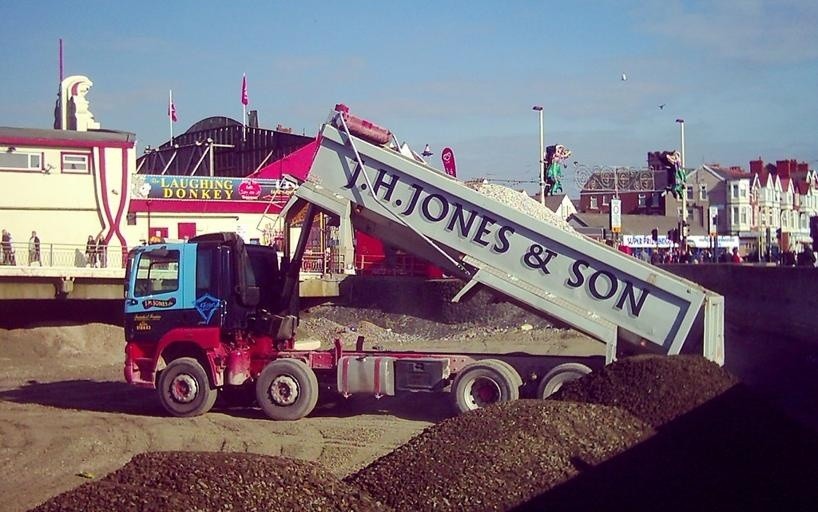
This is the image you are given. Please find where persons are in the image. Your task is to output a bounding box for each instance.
[97,235,107,268]
[637,242,816,267]
[2,229,16,266]
[273,230,285,251]
[86,236,97,267]
[28,231,42,266]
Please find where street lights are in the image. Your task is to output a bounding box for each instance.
[532,108,548,206]
[674,117,686,244]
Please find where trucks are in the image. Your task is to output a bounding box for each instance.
[122,107,725,421]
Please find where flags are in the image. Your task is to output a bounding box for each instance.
[168,100,177,122]
[242,76,248,105]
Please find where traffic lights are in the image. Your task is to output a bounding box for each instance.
[713,217,716,224]
[651,230,656,240]
[774,227,781,241]
